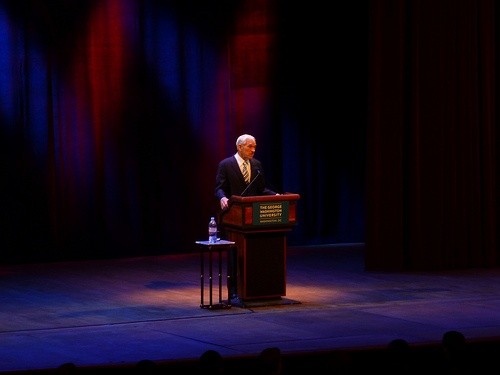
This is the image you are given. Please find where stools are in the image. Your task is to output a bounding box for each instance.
[196,240,236,310]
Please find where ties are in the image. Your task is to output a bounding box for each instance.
[242,163,249,184]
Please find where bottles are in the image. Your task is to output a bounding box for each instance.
[209,217,217,243]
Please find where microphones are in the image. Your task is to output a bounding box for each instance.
[240,169,261,196]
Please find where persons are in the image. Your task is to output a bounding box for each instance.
[217,134,280,307]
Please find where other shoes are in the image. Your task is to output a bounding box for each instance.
[228,295,238,305]
